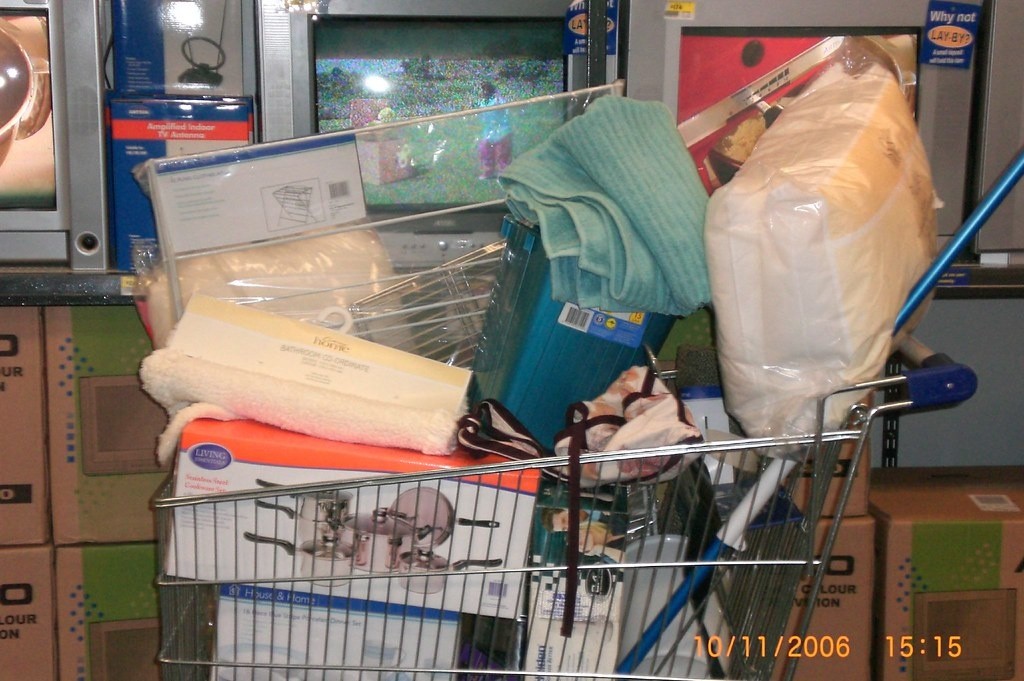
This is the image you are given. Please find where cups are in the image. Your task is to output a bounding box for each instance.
[361,639,406,681]
[616,535,731,681]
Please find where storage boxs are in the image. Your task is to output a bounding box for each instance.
[0,0,1024,681]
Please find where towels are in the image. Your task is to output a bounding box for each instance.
[497,92,722,320]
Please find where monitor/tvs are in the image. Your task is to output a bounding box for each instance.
[629,0,985,270]
[0,0,112,270]
[973,0,1024,269]
[286,0,630,272]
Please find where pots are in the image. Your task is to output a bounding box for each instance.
[243,477,502,594]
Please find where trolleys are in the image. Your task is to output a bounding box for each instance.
[149,287,981,681]
